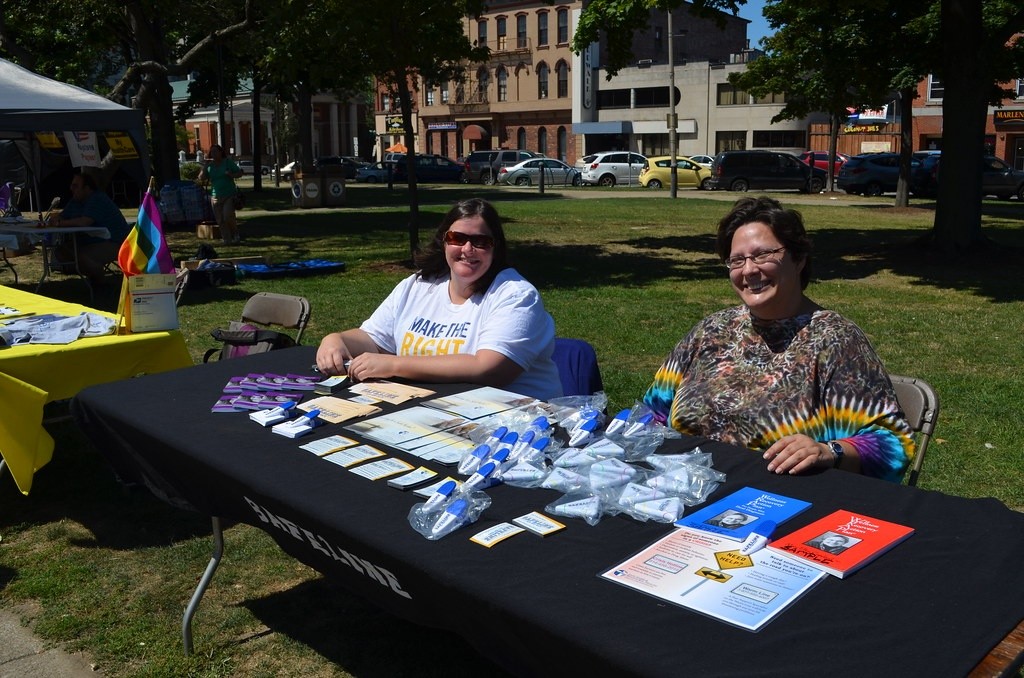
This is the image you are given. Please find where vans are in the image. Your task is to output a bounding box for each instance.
[708,149,828,193]
[464,149,540,186]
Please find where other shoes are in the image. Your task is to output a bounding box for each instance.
[234,231,240,242]
[220,240,231,246]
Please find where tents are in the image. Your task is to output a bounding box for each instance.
[0,57,142,213]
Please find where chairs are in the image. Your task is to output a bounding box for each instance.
[240,291,312,346]
[96,222,136,280]
[888,371,940,488]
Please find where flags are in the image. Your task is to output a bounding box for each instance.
[117,189,176,277]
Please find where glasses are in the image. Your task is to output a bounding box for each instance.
[725,246,788,269]
[442,230,496,250]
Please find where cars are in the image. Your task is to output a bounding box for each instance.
[638,154,719,191]
[395,154,472,184]
[795,151,852,175]
[235,161,270,176]
[582,151,666,187]
[496,158,588,188]
[837,151,921,198]
[355,161,398,184]
[386,153,406,169]
[911,150,941,165]
[520,152,548,158]
[915,154,1024,203]
[271,159,317,182]
[677,155,715,168]
[317,157,370,180]
[575,155,591,168]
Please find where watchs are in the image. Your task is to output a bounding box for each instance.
[820,440,844,468]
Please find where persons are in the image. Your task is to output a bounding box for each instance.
[804,534,850,555]
[704,513,748,530]
[49,171,128,292]
[642,196,917,485]
[316,198,563,403]
[198,145,244,246]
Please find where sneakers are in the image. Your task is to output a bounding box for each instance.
[102,281,116,306]
[94,284,104,304]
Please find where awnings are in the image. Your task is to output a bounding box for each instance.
[462,124,487,140]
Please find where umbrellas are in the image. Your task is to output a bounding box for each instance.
[385,143,407,152]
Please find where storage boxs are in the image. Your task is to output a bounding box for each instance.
[197,224,221,239]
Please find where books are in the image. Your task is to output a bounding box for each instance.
[765,509,915,580]
[673,487,813,542]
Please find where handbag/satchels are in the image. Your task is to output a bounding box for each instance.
[195,244,218,260]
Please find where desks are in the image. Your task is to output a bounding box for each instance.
[56,345,1023,678]
[0,222,112,306]
[1,284,195,495]
